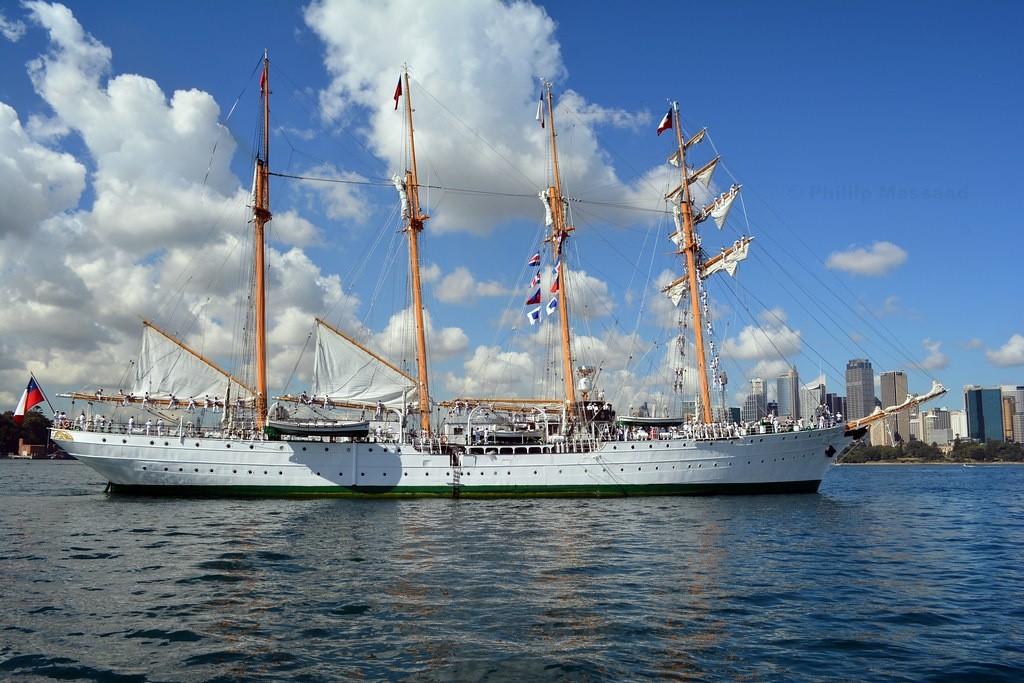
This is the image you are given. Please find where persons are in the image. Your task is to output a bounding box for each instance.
[54,388,842,440]
[673,132,747,393]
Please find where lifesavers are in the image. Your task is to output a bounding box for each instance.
[441,435,448,443]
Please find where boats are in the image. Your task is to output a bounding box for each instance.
[264,402,371,439]
[616,415,685,427]
[51,53,950,504]
[483,424,546,440]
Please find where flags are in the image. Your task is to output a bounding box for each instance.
[525,236,564,327]
[394,74,402,110]
[536,89,545,129]
[259,67,265,98]
[12,377,46,428]
[657,107,672,136]
